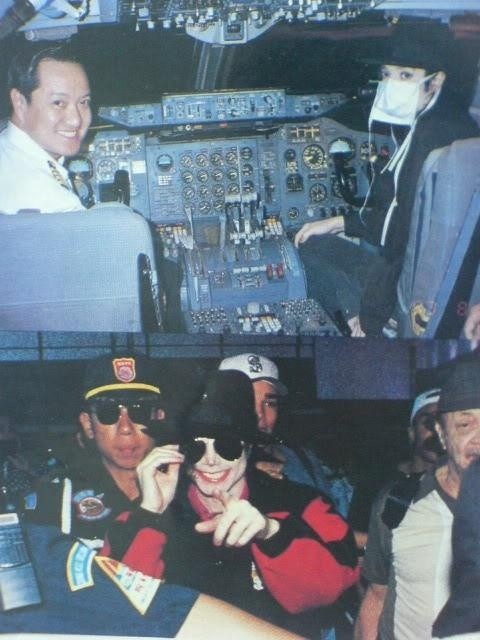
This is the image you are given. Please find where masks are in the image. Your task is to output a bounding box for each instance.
[370,73,437,125]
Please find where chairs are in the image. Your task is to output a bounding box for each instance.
[395,137,478,339]
[1,204,159,336]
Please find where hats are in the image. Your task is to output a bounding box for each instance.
[410,363,480,423]
[141,353,280,443]
[82,351,161,399]
[354,29,459,70]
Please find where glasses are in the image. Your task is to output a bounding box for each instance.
[183,439,243,464]
[97,399,154,424]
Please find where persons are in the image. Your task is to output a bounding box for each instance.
[395,386,443,475]
[1,39,96,212]
[11,349,166,559]
[290,17,478,343]
[461,259,480,355]
[353,357,479,640]
[98,367,363,638]
[217,350,332,500]
